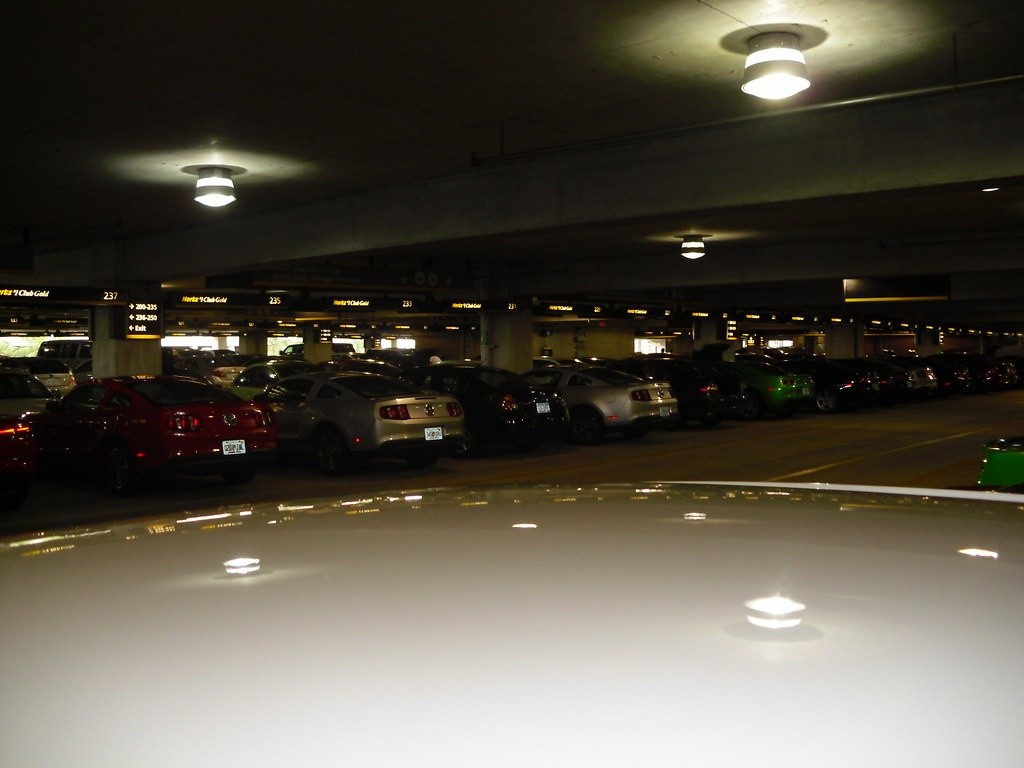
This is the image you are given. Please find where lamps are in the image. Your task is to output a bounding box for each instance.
[193,166,238,208]
[740,31,811,100]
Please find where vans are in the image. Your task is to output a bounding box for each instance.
[39,340,92,372]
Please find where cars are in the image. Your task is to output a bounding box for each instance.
[522,367,678,446]
[603,358,752,429]
[0,366,59,417]
[399,367,570,458]
[24,373,279,498]
[339,359,417,384]
[771,356,885,415]
[164,342,349,402]
[682,355,815,422]
[0,357,78,393]
[864,354,1024,403]
[0,416,35,513]
[252,369,466,478]
